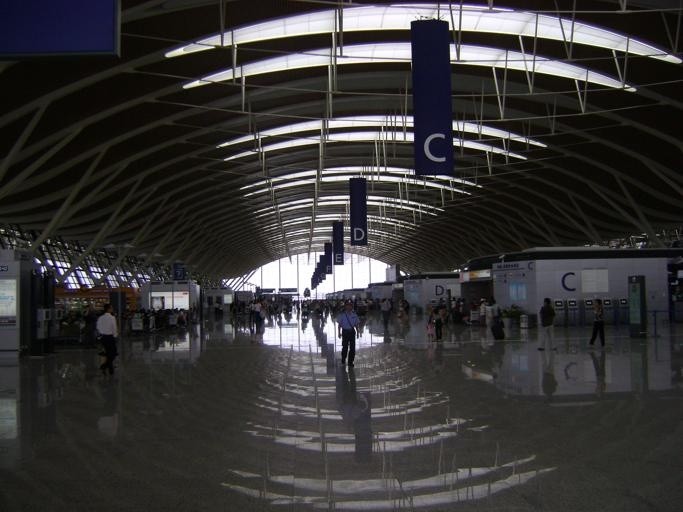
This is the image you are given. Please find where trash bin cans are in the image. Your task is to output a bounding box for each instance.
[520,314,534,329]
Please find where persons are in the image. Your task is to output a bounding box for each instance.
[538,298,555,350]
[587,299,605,347]
